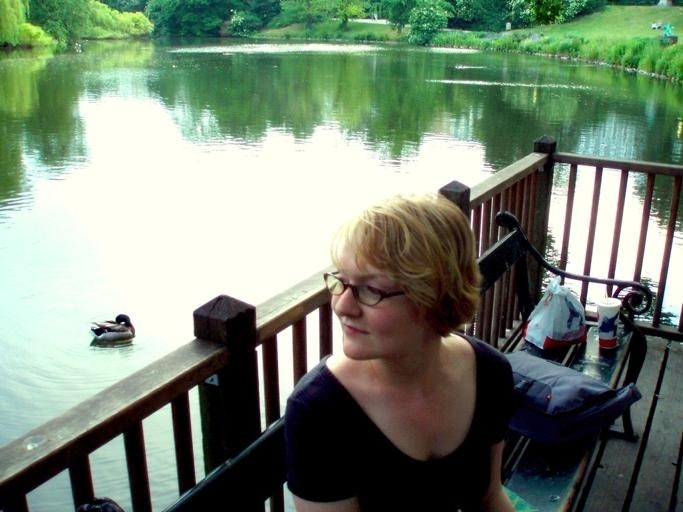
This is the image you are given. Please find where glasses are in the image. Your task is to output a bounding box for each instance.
[321,269,404,307]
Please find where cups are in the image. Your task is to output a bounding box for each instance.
[595,298,618,349]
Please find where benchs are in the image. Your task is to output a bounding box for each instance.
[76,206,655,512]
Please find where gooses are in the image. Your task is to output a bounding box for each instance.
[91,314,136,341]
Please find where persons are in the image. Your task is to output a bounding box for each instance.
[283,195,515,512]
